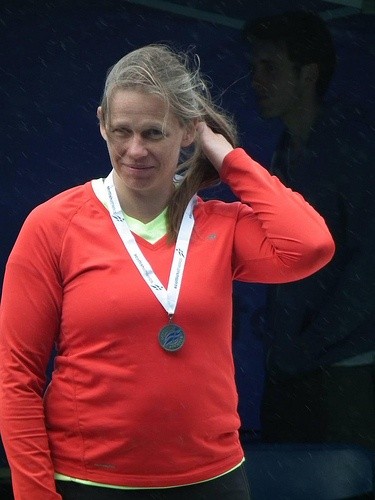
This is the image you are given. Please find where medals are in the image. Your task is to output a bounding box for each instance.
[157,324,186,352]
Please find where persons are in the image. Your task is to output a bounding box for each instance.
[0,45,336,500]
